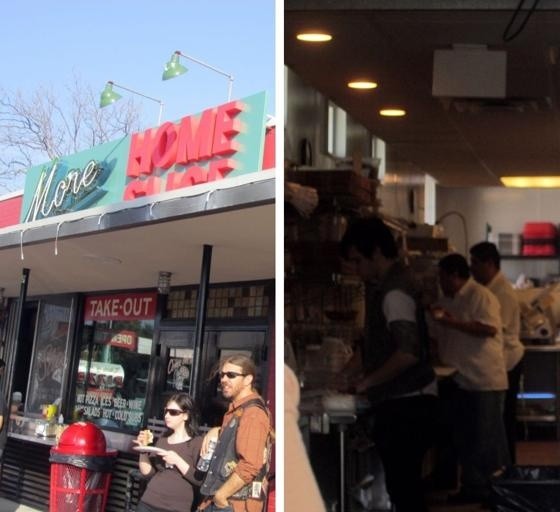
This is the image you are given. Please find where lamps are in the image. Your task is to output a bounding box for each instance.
[97,48,235,126]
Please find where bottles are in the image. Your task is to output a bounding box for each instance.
[196,438,220,473]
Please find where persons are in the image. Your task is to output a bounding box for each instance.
[431,252,508,504]
[130,390,203,512]
[357,223,440,511]
[470,241,524,464]
[197,355,272,512]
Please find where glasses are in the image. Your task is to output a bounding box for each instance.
[163,407,187,415]
[219,371,246,378]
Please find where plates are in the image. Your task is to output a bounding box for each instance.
[130,445,167,455]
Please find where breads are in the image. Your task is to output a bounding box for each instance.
[143,430,153,445]
